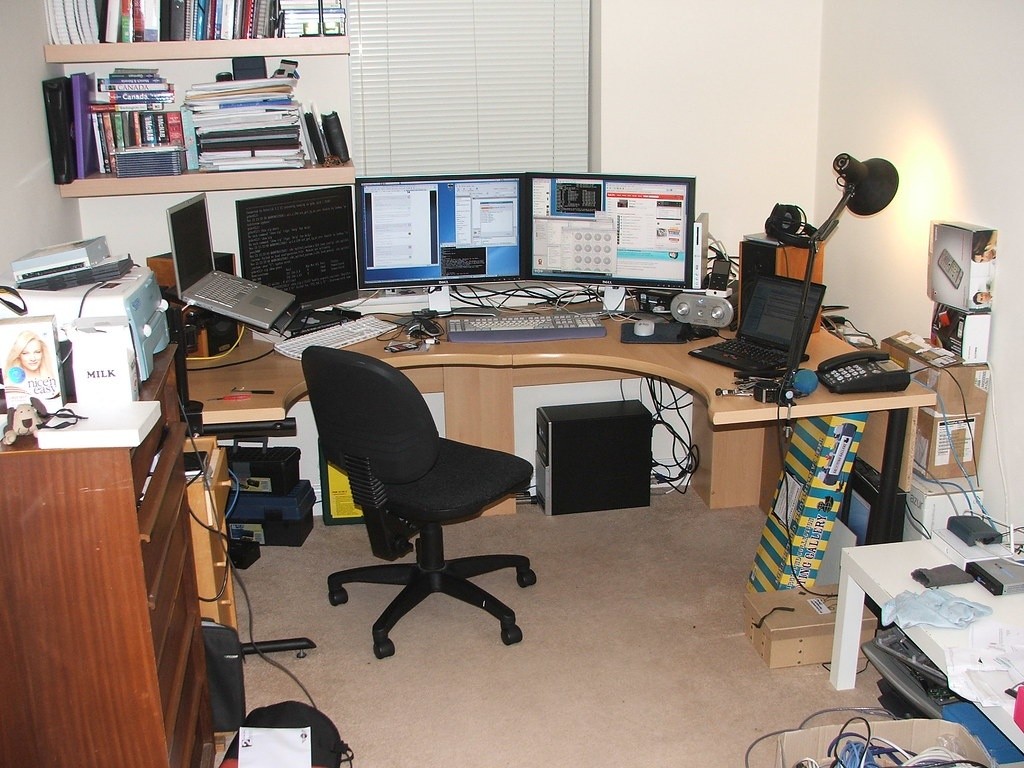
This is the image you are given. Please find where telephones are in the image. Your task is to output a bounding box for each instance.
[816,349,911,393]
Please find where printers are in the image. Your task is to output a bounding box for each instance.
[0,267,172,383]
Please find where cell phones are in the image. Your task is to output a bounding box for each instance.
[384,342,418,353]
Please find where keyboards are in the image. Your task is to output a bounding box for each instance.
[446,315,607,343]
[274,316,397,360]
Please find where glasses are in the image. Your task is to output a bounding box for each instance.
[0,286,27,315]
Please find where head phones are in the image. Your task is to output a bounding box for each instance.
[766,204,818,248]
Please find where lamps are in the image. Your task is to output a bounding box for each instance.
[776,153,899,408]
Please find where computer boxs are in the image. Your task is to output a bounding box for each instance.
[535,399,653,515]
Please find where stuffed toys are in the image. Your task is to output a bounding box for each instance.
[3,396,51,445]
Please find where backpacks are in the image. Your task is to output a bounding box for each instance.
[219,701,354,768]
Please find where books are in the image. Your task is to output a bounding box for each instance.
[42,67,175,185]
[297,98,351,163]
[183,78,307,173]
[46,0,270,44]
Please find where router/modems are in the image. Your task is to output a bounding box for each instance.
[964,556,1024,597]
[929,524,1014,573]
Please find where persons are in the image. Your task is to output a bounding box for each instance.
[972,290,992,307]
[971,230,997,263]
[5,329,60,401]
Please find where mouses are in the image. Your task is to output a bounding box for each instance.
[406,320,443,338]
[633,320,654,335]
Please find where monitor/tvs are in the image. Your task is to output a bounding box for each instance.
[228,172,698,332]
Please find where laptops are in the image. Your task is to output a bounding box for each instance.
[688,272,826,371]
[166,191,296,329]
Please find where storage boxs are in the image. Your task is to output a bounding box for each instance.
[38,400,162,450]
[746,410,869,594]
[743,583,878,669]
[881,330,990,480]
[902,472,984,542]
[218,445,316,547]
[67,317,140,403]
[926,221,998,314]
[931,302,991,364]
[773,719,1001,768]
[0,315,65,414]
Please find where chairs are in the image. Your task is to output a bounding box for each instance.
[300,346,536,659]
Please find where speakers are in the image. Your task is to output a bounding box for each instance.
[738,240,823,334]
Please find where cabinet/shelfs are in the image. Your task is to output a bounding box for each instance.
[45,33,356,198]
[182,436,237,631]
[0,344,216,768]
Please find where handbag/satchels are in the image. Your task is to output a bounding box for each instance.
[200,615,246,732]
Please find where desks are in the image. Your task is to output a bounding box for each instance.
[183,301,937,631]
[829,539,1024,755]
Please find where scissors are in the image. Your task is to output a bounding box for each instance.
[207,393,251,402]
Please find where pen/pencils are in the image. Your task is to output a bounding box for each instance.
[233,389,275,394]
